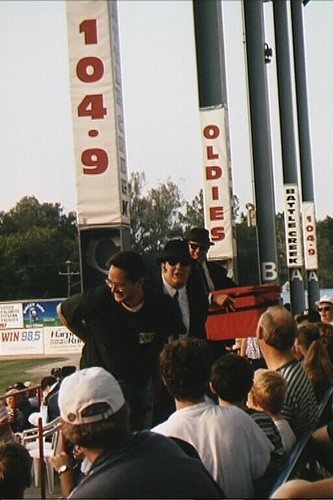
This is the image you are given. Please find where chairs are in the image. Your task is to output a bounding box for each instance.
[21,415,61,494]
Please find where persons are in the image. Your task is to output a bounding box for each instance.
[0,297,333,500]
[56,227,240,485]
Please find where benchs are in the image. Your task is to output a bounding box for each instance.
[252,387,333,500]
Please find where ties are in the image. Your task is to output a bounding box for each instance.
[172,292,179,361]
[199,261,210,293]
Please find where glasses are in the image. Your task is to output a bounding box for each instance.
[318,306,333,312]
[188,243,208,251]
[104,279,131,290]
[165,258,190,267]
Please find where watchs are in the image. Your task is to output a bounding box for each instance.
[58,464,73,475]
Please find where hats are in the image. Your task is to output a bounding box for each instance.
[57,366,126,425]
[315,295,333,307]
[183,227,214,246]
[155,239,191,263]
